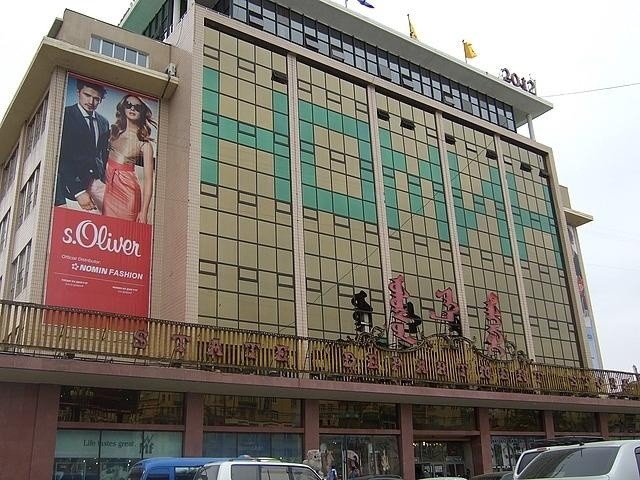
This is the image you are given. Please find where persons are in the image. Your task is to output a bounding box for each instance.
[102,93,158,224]
[326,464,339,480]
[54,79,109,216]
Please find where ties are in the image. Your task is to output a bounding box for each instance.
[86,115,96,148]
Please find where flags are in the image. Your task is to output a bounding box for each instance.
[408,16,420,41]
[464,41,477,59]
[356,0,375,9]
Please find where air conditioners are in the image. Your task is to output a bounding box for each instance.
[163,63,177,77]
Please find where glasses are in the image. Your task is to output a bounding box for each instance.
[124,101,143,112]
